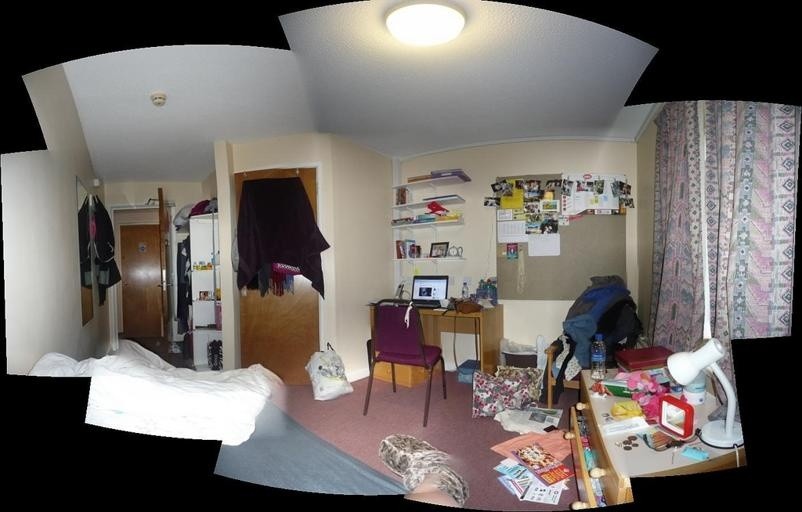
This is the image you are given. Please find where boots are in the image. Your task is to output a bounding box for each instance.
[378,432,470,507]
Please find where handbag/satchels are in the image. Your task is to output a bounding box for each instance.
[303,342,355,400]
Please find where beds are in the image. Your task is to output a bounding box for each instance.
[174,399,411,495]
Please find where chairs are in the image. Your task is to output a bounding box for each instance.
[363,299,447,428]
[544,342,580,409]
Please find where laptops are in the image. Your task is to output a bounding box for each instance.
[399,274,449,309]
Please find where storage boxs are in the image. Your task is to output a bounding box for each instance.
[456,359,480,384]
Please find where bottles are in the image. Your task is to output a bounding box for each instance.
[591,334,606,380]
[462,282,469,299]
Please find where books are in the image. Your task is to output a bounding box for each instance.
[494,441,575,505]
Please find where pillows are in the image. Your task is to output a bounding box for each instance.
[495,365,544,402]
[472,370,539,418]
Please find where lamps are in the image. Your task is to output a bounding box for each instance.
[667,337,743,449]
[383,1,467,47]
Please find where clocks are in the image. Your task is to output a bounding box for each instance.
[449,246,458,255]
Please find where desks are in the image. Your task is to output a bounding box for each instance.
[369,298,503,387]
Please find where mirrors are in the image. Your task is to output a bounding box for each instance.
[76,175,94,329]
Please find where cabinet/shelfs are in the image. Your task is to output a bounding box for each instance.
[189,213,218,371]
[565,366,745,509]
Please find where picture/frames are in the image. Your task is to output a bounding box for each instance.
[430,242,449,257]
